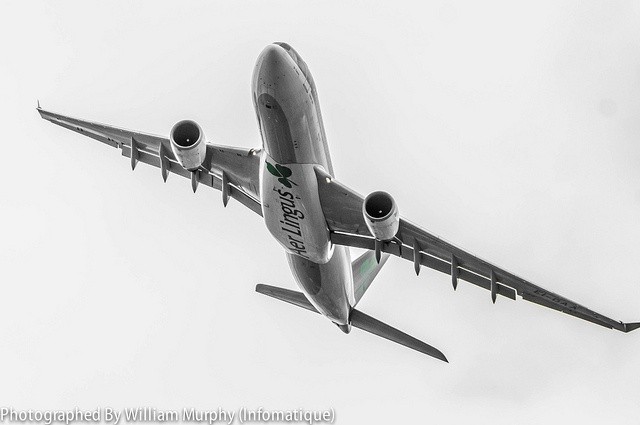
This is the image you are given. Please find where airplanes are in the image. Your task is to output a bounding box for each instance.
[36,41,640,363]
